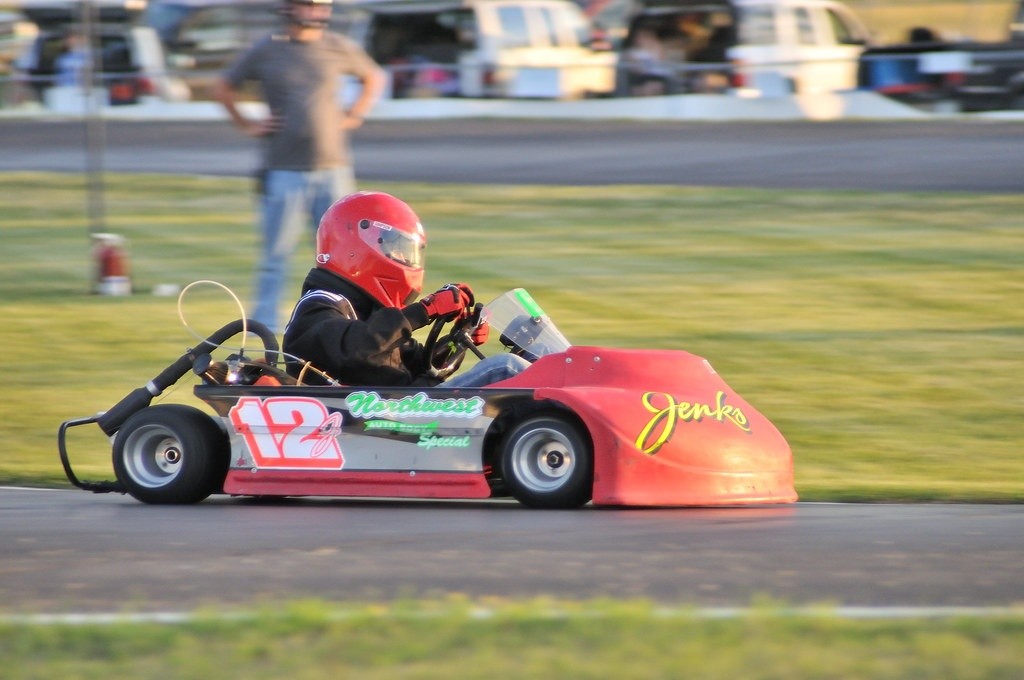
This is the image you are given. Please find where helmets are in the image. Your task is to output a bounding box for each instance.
[315,191,426,311]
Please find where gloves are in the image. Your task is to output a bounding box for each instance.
[419,282,475,323]
[448,303,492,346]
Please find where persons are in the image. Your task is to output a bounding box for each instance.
[56,26,91,88]
[217,0,385,335]
[282,192,489,388]
[572,12,743,99]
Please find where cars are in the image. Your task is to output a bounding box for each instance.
[161,0,279,102]
[11,1,197,103]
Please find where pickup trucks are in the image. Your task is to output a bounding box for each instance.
[857,0,1023,111]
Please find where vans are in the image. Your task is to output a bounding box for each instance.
[614,1,871,100]
[342,2,619,100]
